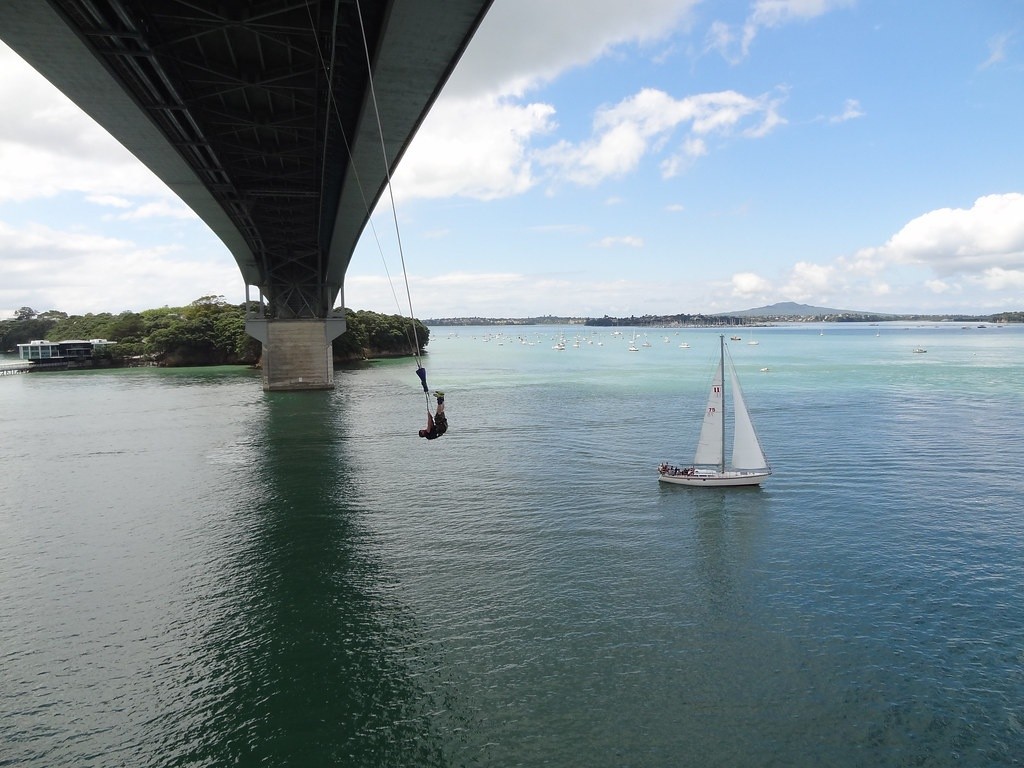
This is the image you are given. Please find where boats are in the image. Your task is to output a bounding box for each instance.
[430,328,690,350]
[760,367,768,372]
[730,336,741,340]
[748,341,759,345]
[978,324,986,328]
[913,349,927,353]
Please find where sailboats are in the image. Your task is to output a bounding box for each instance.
[657,332,773,487]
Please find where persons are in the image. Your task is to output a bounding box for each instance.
[658,462,695,477]
[419,390,448,439]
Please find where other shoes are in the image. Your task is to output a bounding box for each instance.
[433,391,445,399]
